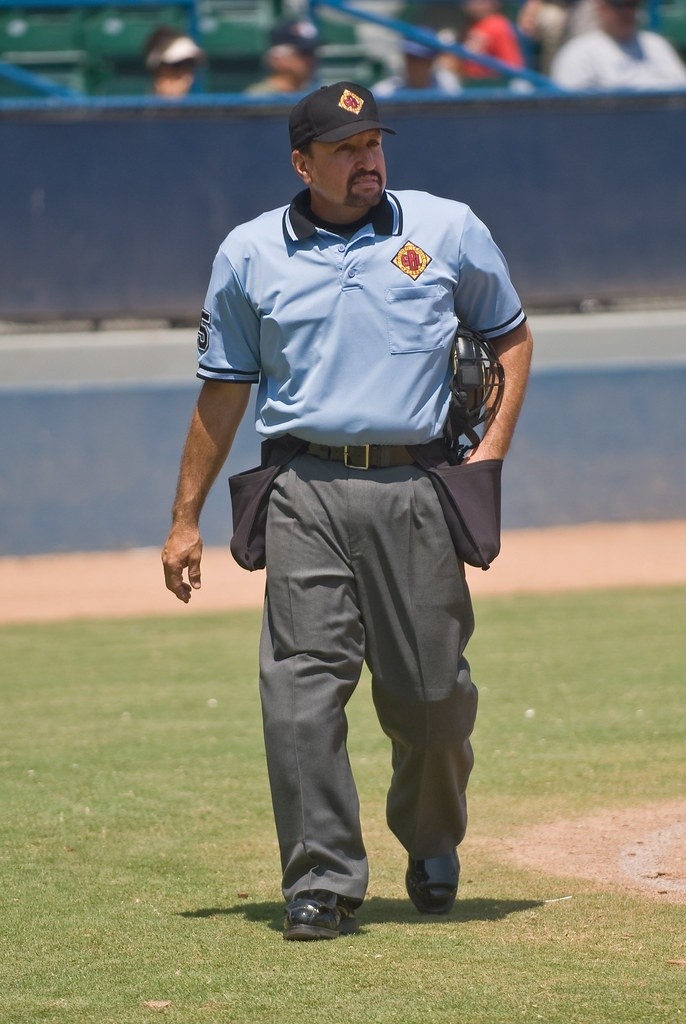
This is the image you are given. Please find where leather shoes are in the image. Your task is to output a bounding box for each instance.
[406,849,460,914]
[283,891,358,940]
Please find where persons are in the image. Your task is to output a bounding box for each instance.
[160,78,533,939]
[137,0,686,95]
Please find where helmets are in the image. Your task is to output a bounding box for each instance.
[446,322,484,437]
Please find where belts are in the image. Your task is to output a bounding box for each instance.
[284,433,416,470]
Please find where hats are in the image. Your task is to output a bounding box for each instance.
[269,18,329,49]
[289,82,397,150]
[144,34,201,67]
